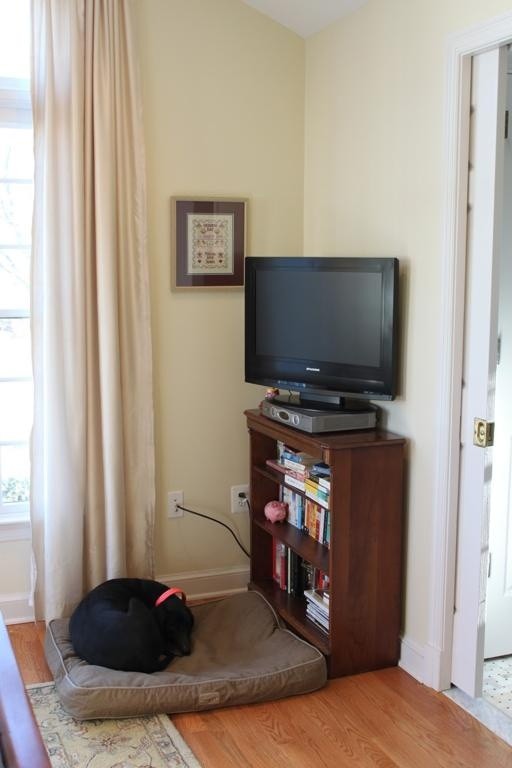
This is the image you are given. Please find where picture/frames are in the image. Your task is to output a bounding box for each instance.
[169,196,249,290]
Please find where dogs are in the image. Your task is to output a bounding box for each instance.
[69,578,194,672]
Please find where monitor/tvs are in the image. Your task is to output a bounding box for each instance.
[245,255,399,412]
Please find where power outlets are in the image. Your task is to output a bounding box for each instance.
[230,485,250,513]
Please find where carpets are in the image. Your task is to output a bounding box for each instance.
[25,680,201,768]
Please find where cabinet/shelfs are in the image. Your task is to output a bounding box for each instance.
[244,408,406,677]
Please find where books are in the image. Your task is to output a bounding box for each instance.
[264,440,331,638]
